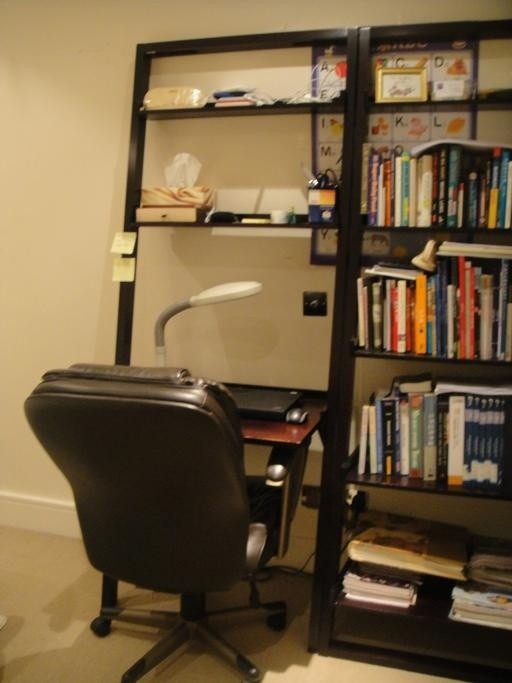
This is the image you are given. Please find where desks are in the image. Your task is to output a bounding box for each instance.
[212,384,328,561]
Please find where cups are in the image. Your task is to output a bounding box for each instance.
[270,210,288,224]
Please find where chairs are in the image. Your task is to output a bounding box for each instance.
[21,360,290,683]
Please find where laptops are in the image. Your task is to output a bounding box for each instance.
[212,382,304,422]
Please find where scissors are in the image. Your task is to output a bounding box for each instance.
[315,169,337,189]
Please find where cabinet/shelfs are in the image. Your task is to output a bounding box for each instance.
[86,27,358,654]
[317,19,512,683]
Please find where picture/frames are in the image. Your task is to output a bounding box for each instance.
[374,65,428,105]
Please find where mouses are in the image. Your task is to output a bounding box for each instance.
[286,407,309,422]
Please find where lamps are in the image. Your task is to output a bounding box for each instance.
[150,281,264,370]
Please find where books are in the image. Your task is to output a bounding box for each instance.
[361,141,512,231]
[340,521,512,631]
[136,206,211,225]
[356,241,512,363]
[356,373,511,491]
